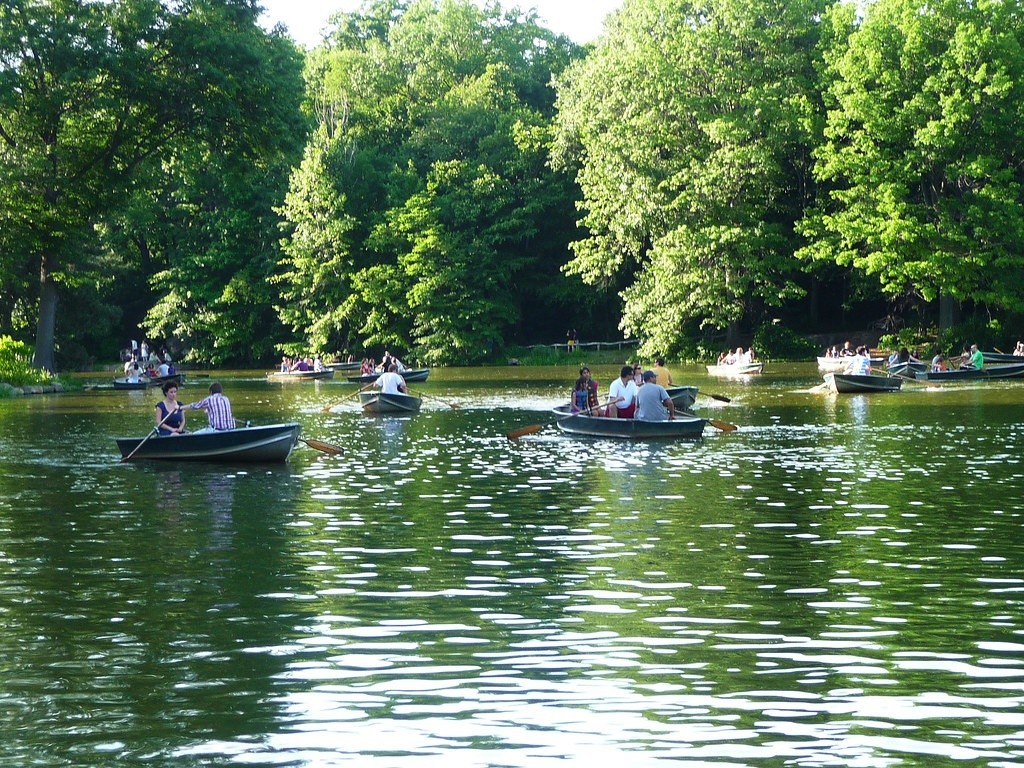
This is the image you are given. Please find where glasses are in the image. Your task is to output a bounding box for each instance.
[636,367,641,369]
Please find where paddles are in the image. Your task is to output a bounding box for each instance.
[234,417,344,454]
[675,410,738,433]
[321,381,376,413]
[507,395,626,440]
[872,367,941,388]
[670,384,731,403]
[84,373,125,392]
[809,382,826,393]
[185,374,209,378]
[598,393,610,397]
[119,407,177,464]
[405,386,462,408]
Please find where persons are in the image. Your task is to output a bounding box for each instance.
[566,323,576,353]
[717,346,754,366]
[840,339,856,357]
[633,371,676,421]
[931,349,947,372]
[951,343,984,369]
[360,349,407,395]
[347,354,353,363]
[844,344,871,376]
[1013,341,1024,356]
[570,366,603,418]
[124,338,176,384]
[175,383,236,434]
[155,382,190,436]
[887,347,922,368]
[280,353,325,373]
[630,363,645,387]
[650,358,672,389]
[605,366,638,418]
[825,345,839,358]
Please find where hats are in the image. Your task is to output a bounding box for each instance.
[643,371,658,380]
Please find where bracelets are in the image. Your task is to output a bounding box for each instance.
[179,405,181,410]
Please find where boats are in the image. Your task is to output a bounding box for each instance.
[552,403,707,438]
[112,373,187,391]
[980,352,1024,363]
[888,362,928,380]
[662,385,700,410]
[347,369,429,382]
[913,365,1024,381]
[817,357,854,370]
[266,370,334,383]
[356,391,423,412]
[823,372,902,394]
[704,362,764,376]
[308,362,361,370]
[116,423,303,464]
